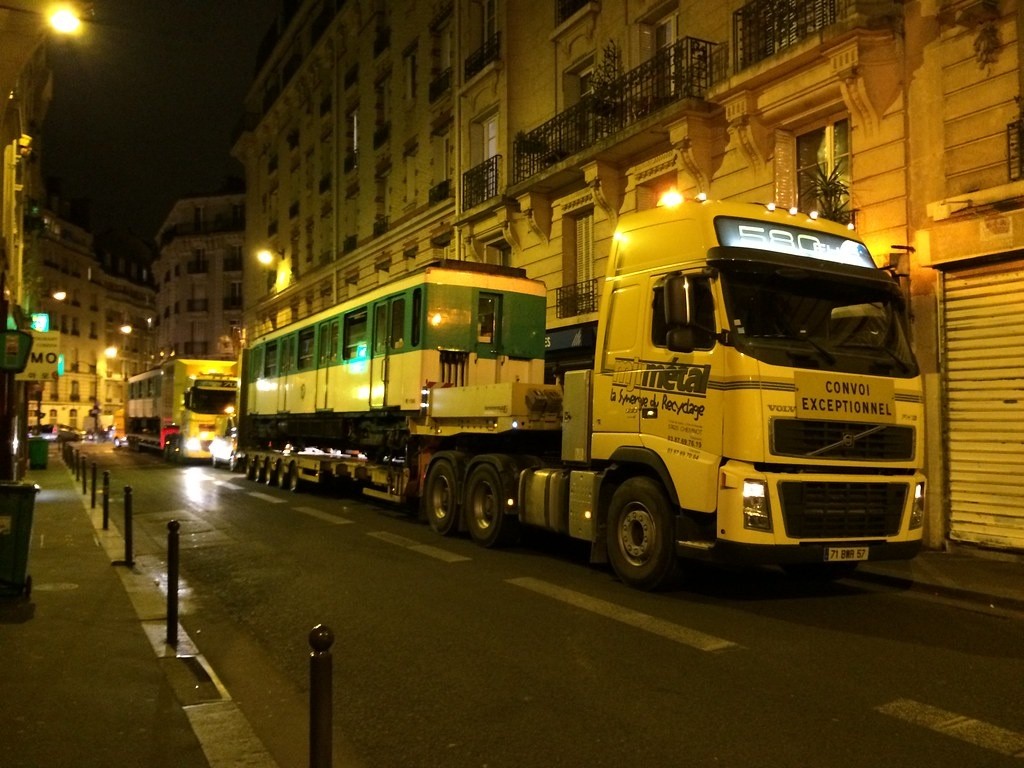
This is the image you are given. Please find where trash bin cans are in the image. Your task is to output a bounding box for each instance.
[29,438,49,470]
[0,482,42,623]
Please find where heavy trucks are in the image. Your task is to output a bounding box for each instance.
[234,196,927,587]
[124,357,240,465]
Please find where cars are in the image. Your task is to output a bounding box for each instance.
[109,424,128,447]
[208,414,243,472]
[38,423,82,442]
[85,429,109,441]
[27,425,40,437]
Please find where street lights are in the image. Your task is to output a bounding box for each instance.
[104,346,145,372]
[74,360,98,429]
[22,290,66,470]
[121,325,157,365]
